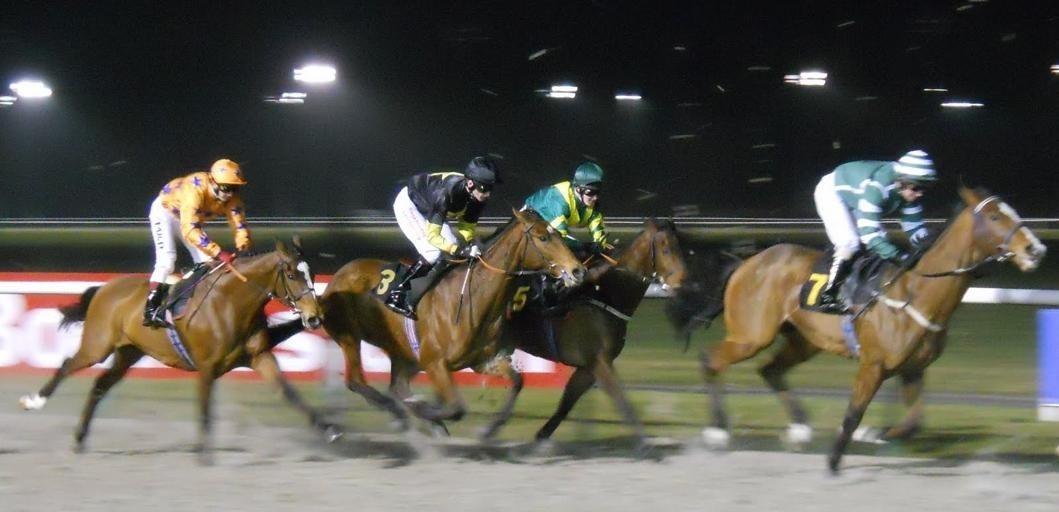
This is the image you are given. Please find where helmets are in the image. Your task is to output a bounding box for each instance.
[572,161,606,191]
[892,146,940,183]
[211,158,248,187]
[465,156,500,184]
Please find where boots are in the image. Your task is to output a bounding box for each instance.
[818,260,850,314]
[385,260,426,319]
[143,290,169,326]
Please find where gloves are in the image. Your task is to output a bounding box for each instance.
[220,250,234,263]
[463,245,482,260]
[589,239,602,255]
[898,246,913,263]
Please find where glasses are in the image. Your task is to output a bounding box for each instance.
[903,182,932,194]
[472,181,489,192]
[216,185,236,192]
[577,187,598,197]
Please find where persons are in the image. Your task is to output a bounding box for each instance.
[386,155,499,321]
[520,162,616,315]
[812,148,938,315]
[140,159,252,328]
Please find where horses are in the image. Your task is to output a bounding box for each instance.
[307,208,588,443]
[668,183,1045,476]
[20,233,343,464]
[518,217,705,450]
